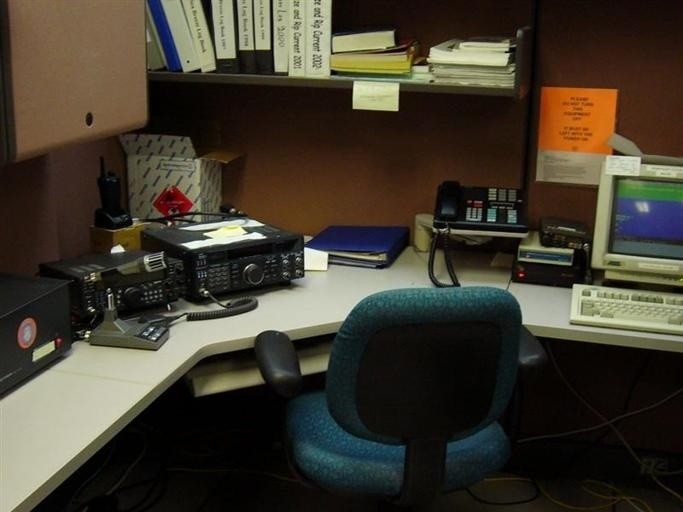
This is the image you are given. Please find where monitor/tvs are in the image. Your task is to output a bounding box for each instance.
[590,154,682,286]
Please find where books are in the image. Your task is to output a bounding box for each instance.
[329,24,515,90]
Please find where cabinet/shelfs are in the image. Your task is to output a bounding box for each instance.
[135,25,534,100]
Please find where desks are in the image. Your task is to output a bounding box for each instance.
[0,238,683,506]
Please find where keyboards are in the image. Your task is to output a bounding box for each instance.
[569,281,683,335]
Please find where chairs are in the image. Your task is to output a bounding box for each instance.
[254,286,546,506]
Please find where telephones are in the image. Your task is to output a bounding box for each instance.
[94,156,133,229]
[433,180,530,232]
